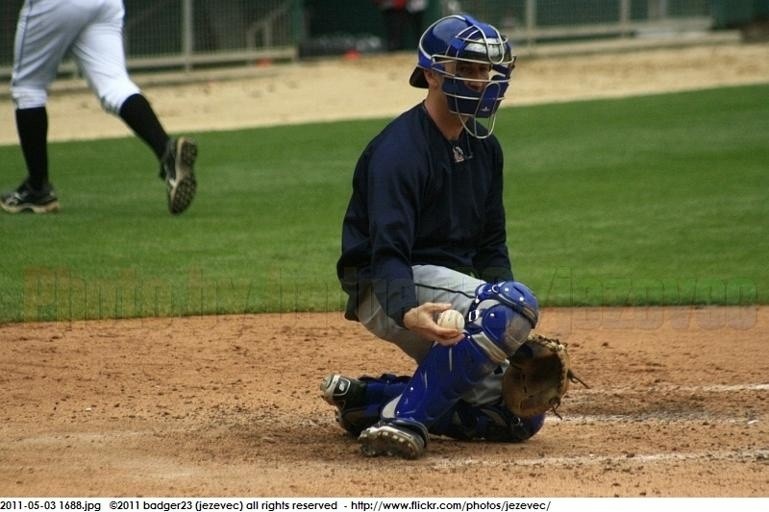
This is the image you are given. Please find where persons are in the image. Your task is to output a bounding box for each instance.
[0,0,198,215]
[316,15,590,460]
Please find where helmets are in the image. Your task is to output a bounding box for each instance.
[409,15,516,140]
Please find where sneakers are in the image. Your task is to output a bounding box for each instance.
[159,137,197,213]
[358,419,426,460]
[321,373,368,434]
[0,182,59,214]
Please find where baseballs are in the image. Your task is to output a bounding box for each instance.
[436,309,465,337]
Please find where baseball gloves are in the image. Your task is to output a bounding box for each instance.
[501,332,591,420]
